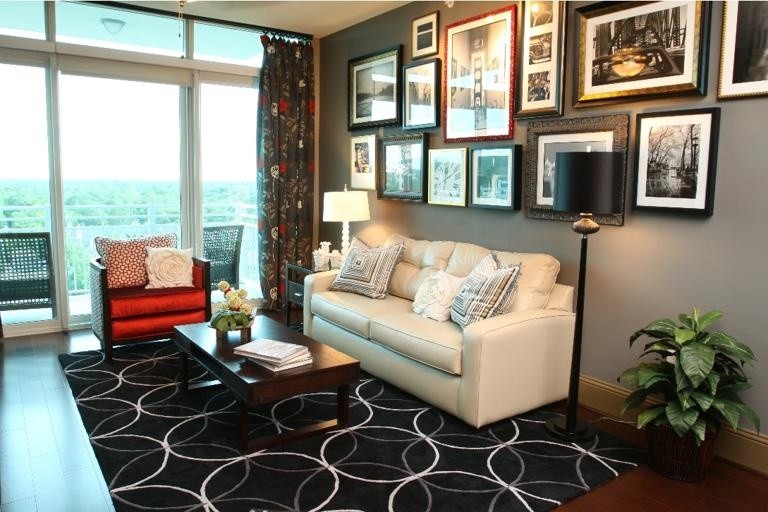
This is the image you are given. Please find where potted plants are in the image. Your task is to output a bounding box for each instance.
[617,306,760,483]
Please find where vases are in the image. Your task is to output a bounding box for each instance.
[207,316,256,342]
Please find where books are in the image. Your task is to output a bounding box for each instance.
[231,338,305,363]
[244,358,314,372]
[256,351,312,367]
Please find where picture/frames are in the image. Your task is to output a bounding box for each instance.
[376,132,430,205]
[349,129,379,192]
[427,147,467,208]
[347,43,405,132]
[443,3,518,144]
[403,58,441,131]
[573,1,713,109]
[630,107,722,219]
[467,144,523,212]
[715,2,765,102]
[513,1,569,121]
[523,112,630,225]
[411,10,441,61]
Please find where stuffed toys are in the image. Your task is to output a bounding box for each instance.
[217,281,252,315]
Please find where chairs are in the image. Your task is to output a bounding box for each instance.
[203,224,244,291]
[88,233,215,361]
[0,232,57,320]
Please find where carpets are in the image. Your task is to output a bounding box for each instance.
[57,320,641,512]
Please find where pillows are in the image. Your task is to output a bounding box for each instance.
[327,236,402,302]
[410,270,463,324]
[142,246,196,289]
[93,233,177,289]
[450,253,522,330]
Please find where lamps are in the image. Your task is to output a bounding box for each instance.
[99,18,124,33]
[321,183,371,264]
[543,151,627,441]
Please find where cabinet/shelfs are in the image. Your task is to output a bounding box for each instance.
[286,261,333,326]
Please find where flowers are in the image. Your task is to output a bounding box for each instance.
[210,280,253,332]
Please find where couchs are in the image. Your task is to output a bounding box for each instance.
[302,235,576,430]
